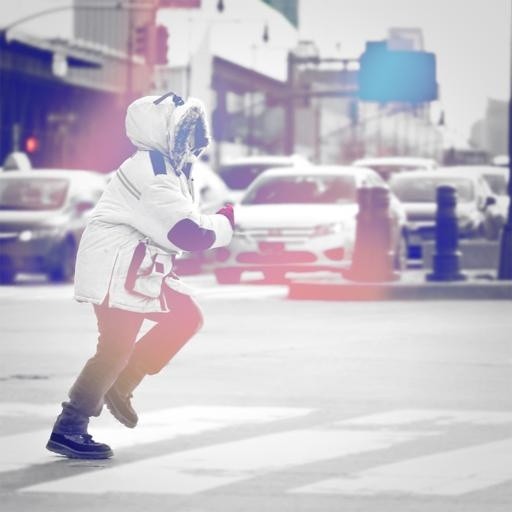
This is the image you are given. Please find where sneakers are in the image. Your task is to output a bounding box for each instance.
[47,433,113,459]
[104,388,137,427]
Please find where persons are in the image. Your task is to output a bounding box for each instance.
[45,90,245,462]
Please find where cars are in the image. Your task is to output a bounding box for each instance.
[83,161,233,267]
[0,169,107,281]
[206,165,411,284]
[207,156,318,263]
[354,155,511,258]
